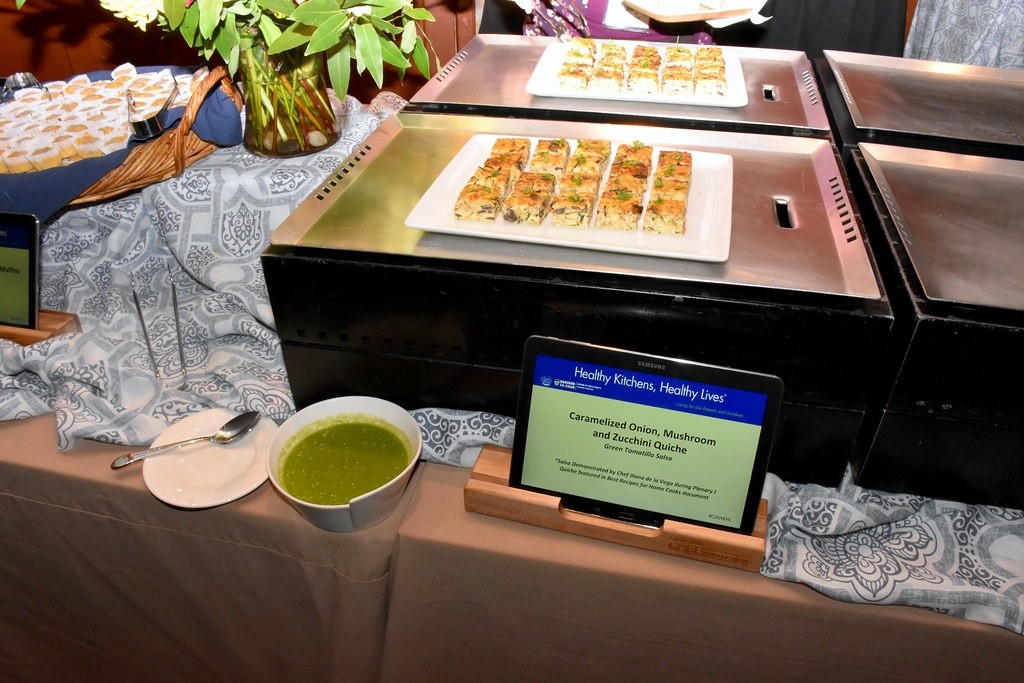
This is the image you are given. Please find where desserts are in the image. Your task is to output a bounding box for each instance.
[454,138,692,235]
[0,61,211,173]
[558,36,727,101]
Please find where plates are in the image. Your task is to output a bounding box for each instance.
[143,406,282,508]
[402,133,733,264]
[525,37,749,109]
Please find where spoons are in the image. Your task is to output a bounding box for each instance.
[109,410,263,470]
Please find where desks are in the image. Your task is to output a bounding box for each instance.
[0,336,1023,681]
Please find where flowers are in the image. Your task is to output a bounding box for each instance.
[100,0,445,155]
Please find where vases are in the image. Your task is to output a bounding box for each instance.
[234,16,342,159]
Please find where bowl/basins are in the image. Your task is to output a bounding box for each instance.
[266,396,423,533]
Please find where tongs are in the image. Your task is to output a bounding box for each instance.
[124,74,180,139]
